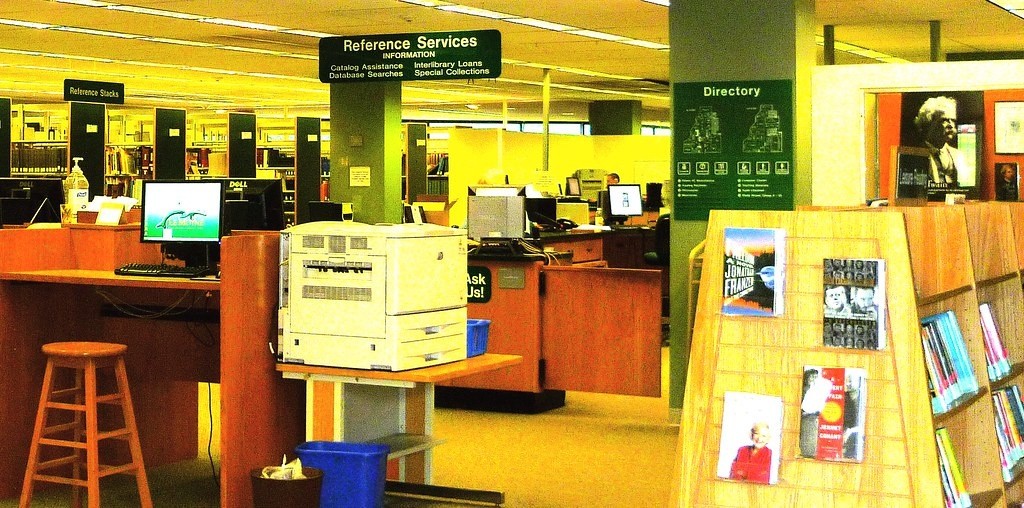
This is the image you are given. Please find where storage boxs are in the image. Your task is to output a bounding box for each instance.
[412,196,459,227]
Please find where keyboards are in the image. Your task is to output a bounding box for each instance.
[115,263,211,277]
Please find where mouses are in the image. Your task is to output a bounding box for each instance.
[216,270,221,278]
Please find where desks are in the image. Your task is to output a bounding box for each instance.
[0,224,306,508]
[428,224,669,415]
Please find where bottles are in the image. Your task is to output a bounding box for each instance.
[594,208,604,226]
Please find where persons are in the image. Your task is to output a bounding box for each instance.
[905,94,974,188]
[996,163,1018,201]
[728,422,774,485]
[587,173,621,204]
[823,259,879,349]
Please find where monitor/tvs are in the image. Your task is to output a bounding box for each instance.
[0,177,64,224]
[566,177,581,196]
[476,187,517,196]
[525,198,589,226]
[140,179,286,267]
[608,184,643,224]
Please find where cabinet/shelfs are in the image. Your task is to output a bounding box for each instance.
[256,167,295,215]
[276,353,523,508]
[667,201,1024,508]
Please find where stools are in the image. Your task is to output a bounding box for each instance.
[19,341,154,508]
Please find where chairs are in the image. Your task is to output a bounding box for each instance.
[644,213,670,266]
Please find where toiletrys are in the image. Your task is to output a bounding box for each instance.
[63,156,89,224]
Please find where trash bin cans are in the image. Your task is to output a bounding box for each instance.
[296,441,391,508]
[250,468,323,508]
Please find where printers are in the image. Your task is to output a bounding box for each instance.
[276,220,468,371]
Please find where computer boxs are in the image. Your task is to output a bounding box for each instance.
[469,196,525,238]
[309,201,354,222]
[597,191,628,222]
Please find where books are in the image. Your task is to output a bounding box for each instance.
[185,147,227,180]
[935,427,973,508]
[427,180,447,195]
[104,146,154,175]
[821,256,888,350]
[105,176,144,208]
[11,147,67,173]
[991,384,1024,482]
[979,304,1013,382]
[427,153,449,176]
[920,309,979,414]
[256,148,295,227]
[721,228,787,317]
[716,390,785,485]
[799,364,868,462]
